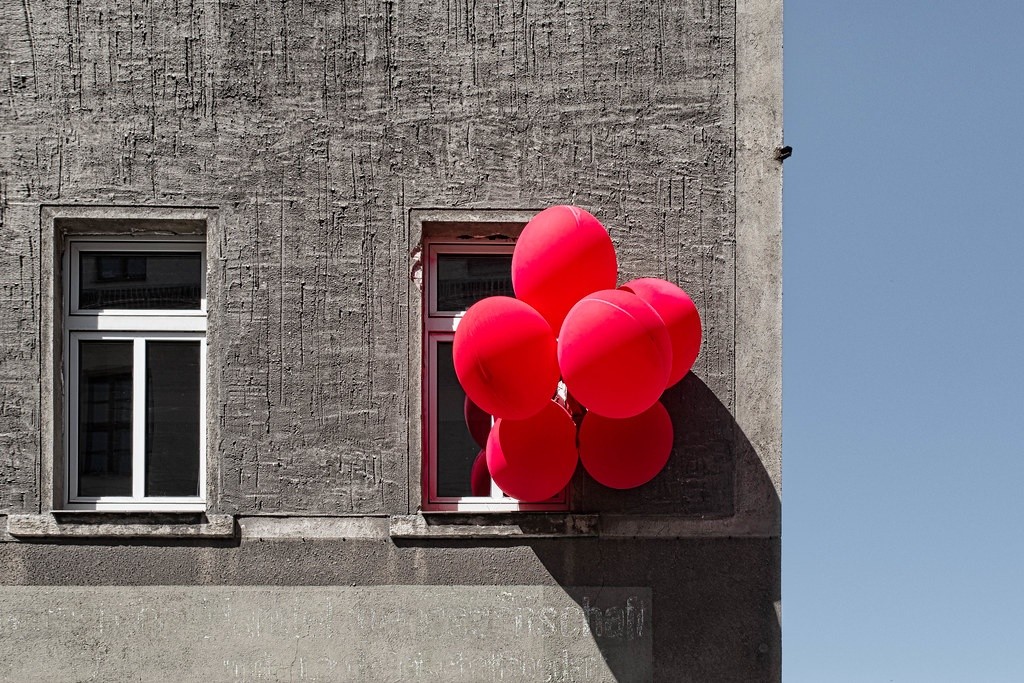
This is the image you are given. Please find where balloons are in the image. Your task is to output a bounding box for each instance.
[451,204,702,504]
